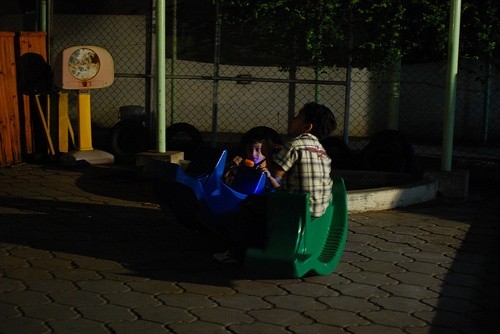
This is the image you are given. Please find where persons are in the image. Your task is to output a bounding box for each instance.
[221,134,283,193]
[260,102,337,218]
